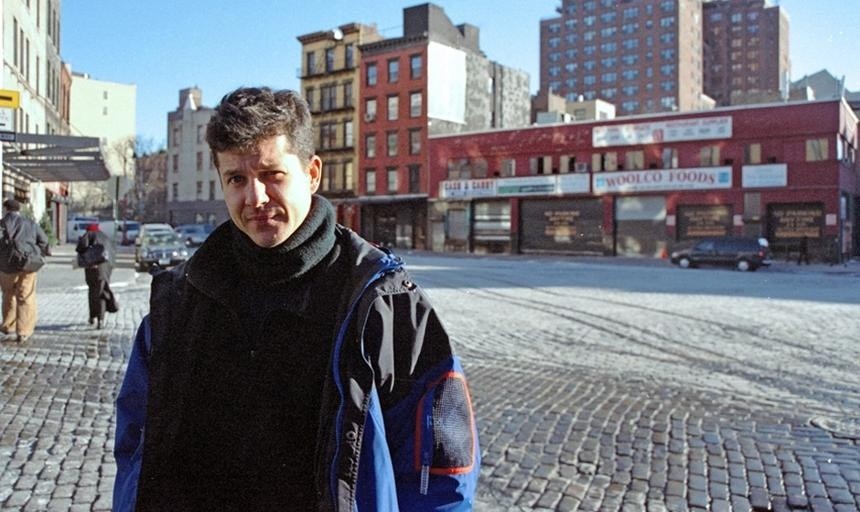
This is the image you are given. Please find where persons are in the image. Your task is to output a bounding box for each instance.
[75,224,116,330]
[112,86,483,511]
[1,197,49,342]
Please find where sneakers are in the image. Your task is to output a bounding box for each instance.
[90,317,105,329]
[3,333,28,345]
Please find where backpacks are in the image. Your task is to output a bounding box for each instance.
[78,243,110,267]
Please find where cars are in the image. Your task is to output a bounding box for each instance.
[66,216,215,268]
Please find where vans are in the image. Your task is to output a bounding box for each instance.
[670,235,772,272]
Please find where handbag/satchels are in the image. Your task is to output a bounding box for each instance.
[7,241,45,271]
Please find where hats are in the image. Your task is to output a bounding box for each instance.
[3,200,20,211]
[86,223,101,232]
